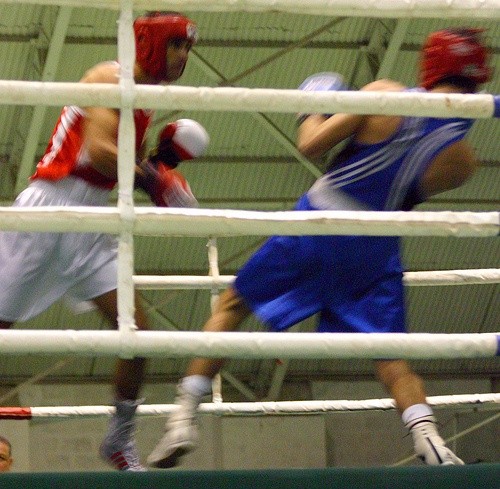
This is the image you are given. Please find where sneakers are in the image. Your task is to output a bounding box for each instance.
[146,425,199,468]
[99,436,146,472]
[415,435,466,467]
[145,422,196,467]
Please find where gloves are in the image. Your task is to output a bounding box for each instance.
[139,157,200,210]
[296,72,349,126]
[159,117,211,170]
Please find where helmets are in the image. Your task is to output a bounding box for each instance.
[422,25,492,89]
[133,15,197,80]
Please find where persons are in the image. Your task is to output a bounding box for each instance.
[0,436,14,471]
[0,10,211,471]
[146,25,496,470]
